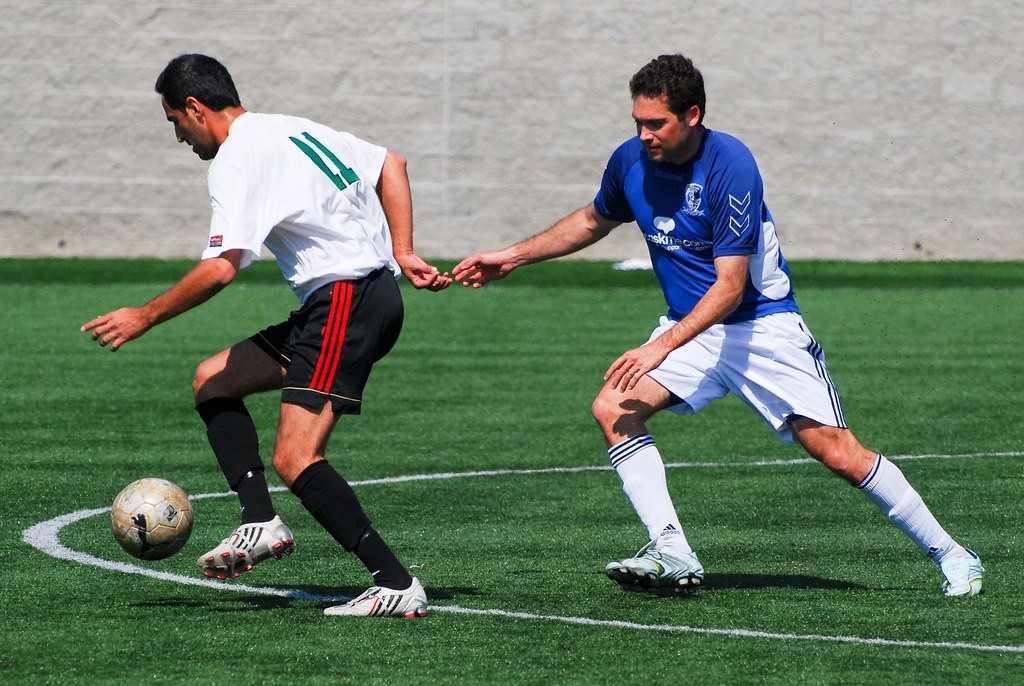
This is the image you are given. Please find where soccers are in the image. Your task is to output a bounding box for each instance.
[109,478,195,560]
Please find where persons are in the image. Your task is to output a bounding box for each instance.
[79,52,452,616]
[452,53,984,597]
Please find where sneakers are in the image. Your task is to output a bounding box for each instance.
[195,512,296,580]
[941,546,984,598]
[604,538,705,596]
[322,576,428,618]
